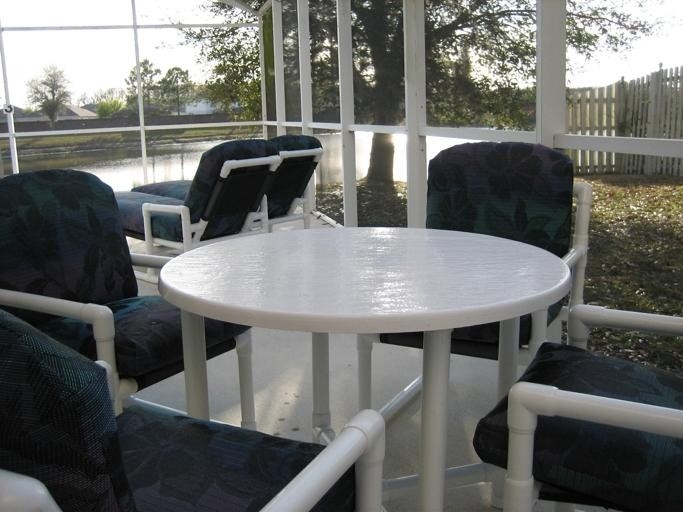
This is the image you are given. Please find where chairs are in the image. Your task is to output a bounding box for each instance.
[131,134,324,233]
[0,308,387,512]
[473,305,683,512]
[0,170,254,418]
[113,139,283,284]
[427,140,593,360]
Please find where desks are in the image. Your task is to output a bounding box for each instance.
[159,227,573,512]
